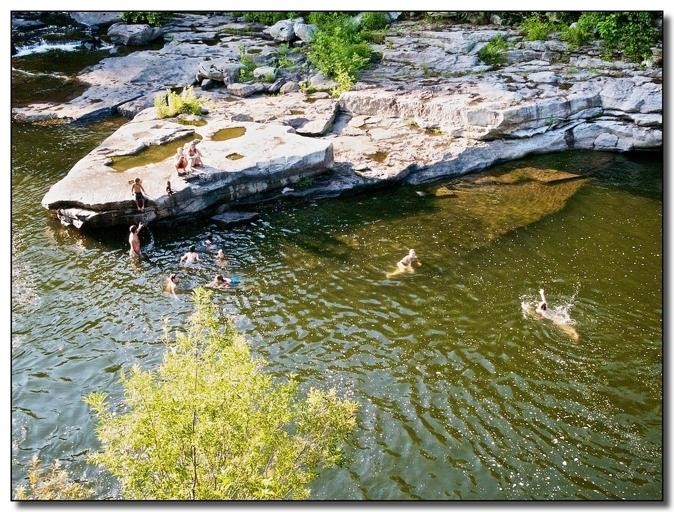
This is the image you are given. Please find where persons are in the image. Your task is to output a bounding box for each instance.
[127,221,145,257]
[165,181,174,196]
[187,142,204,168]
[384,248,423,279]
[165,274,182,303]
[173,147,188,176]
[214,249,228,269]
[203,233,215,246]
[130,178,147,213]
[519,287,580,344]
[202,273,242,290]
[179,244,199,265]
